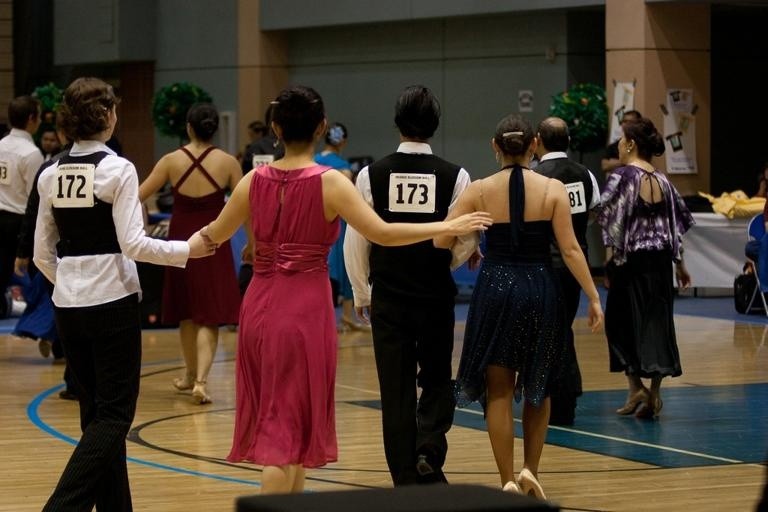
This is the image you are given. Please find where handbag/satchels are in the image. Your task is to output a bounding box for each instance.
[734,261,768,313]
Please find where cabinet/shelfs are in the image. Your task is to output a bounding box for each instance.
[670,211,751,297]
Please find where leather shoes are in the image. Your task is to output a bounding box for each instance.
[418,453,450,487]
[39,339,51,359]
[59,388,75,399]
[53,338,64,359]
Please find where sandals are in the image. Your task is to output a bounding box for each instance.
[174,373,194,390]
[192,379,213,404]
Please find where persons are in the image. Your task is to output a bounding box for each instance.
[312,122,364,333]
[530,118,602,424]
[40,110,63,158]
[343,87,479,487]
[601,109,657,170]
[32,77,219,511]
[199,86,494,496]
[599,120,696,421]
[234,95,286,176]
[141,99,244,402]
[432,113,605,502]
[752,163,768,233]
[0,96,45,319]
[13,104,84,359]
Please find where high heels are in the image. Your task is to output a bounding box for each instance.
[518,468,545,501]
[503,480,519,493]
[639,398,663,419]
[339,316,360,331]
[616,387,649,415]
